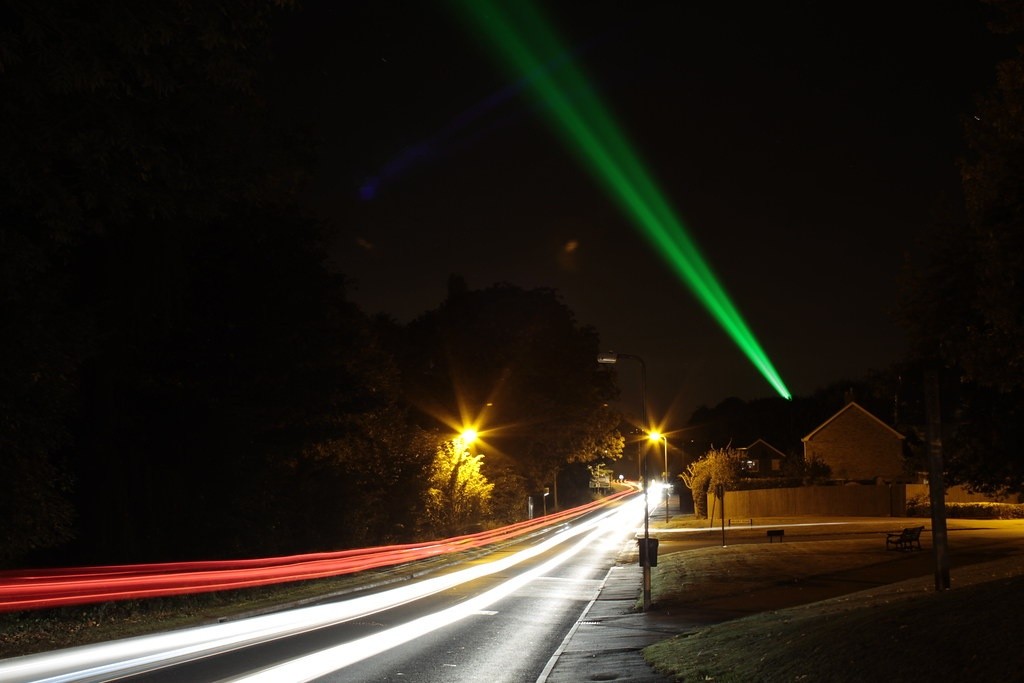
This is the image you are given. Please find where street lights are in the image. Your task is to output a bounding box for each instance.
[597,350,659,611]
[648,431,669,523]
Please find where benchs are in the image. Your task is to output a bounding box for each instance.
[886,525,926,551]
